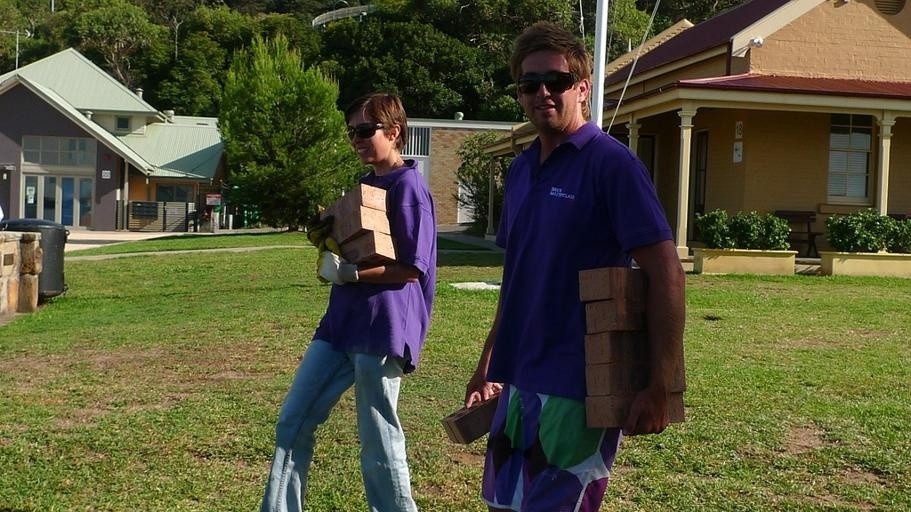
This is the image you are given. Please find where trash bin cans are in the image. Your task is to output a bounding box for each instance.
[226,214,233,229]
[0,218,69,298]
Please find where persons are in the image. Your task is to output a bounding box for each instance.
[466,20,684,512]
[265,93,438,512]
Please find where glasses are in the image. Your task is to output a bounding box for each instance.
[517,71,576,96]
[346,122,384,141]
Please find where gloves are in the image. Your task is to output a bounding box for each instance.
[306,214,335,249]
[317,250,360,287]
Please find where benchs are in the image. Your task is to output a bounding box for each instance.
[773,210,824,258]
